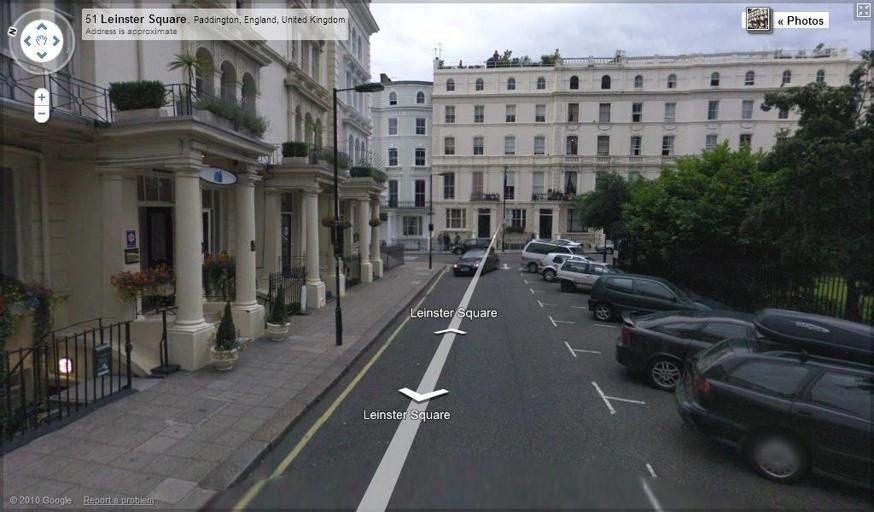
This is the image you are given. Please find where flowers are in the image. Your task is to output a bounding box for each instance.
[110,249,236,303]
[0,274,70,362]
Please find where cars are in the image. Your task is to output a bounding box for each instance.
[595,239,623,253]
[454,249,500,277]
[448,238,492,254]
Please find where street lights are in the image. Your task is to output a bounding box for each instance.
[331,82,386,351]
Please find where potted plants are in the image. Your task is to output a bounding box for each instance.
[264,283,291,343]
[317,145,389,185]
[281,141,309,165]
[168,48,208,116]
[106,80,168,123]
[194,97,268,140]
[209,302,239,371]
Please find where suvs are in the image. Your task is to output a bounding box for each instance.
[522,239,585,271]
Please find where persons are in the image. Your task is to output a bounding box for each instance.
[436,230,461,252]
